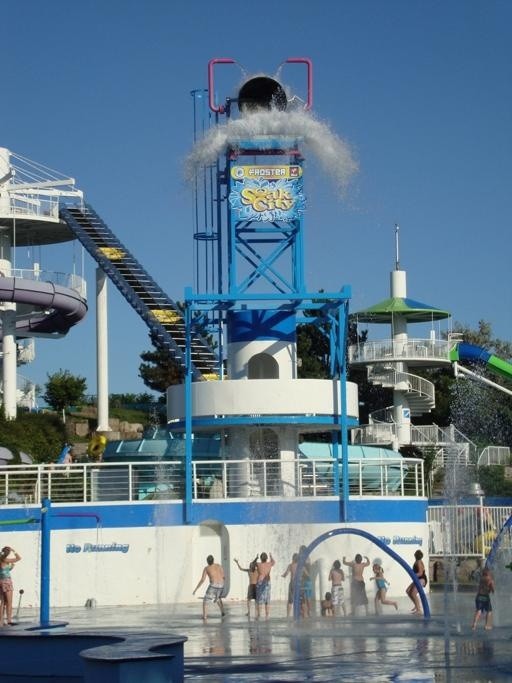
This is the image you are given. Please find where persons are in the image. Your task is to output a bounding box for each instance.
[469,565,496,631]
[280,552,312,618]
[297,544,316,618]
[64,452,72,464]
[328,559,347,617]
[320,591,333,618]
[342,553,372,617]
[191,553,226,622]
[404,548,427,614]
[251,551,276,617]
[368,563,398,616]
[1,546,22,627]
[233,557,259,617]
[200,621,496,674]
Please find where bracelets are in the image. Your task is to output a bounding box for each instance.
[14,551,17,555]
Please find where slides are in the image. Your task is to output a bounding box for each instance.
[0,276,88,342]
[459,342,512,376]
[102,423,409,499]
